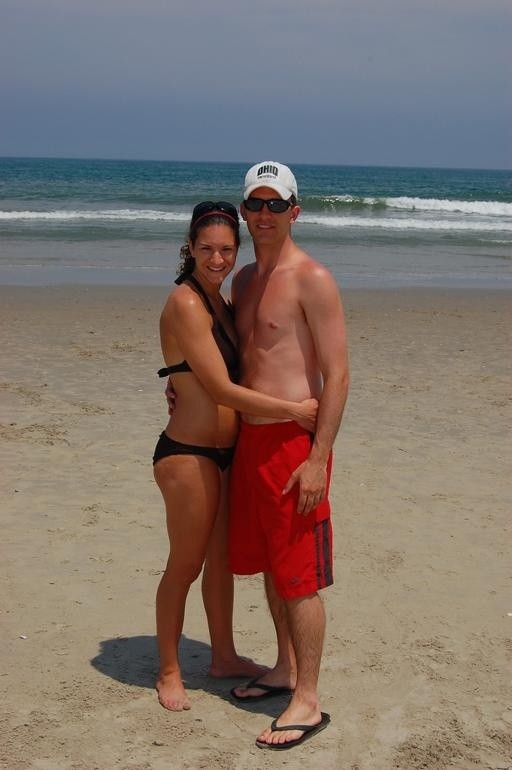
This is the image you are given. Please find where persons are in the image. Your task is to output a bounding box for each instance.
[165,159,351,752]
[152,200,321,712]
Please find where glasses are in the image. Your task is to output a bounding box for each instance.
[243,197,294,214]
[191,201,237,225]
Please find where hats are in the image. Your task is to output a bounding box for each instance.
[242,161,298,201]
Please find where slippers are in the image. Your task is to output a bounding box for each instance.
[229,671,299,701]
[254,710,331,751]
